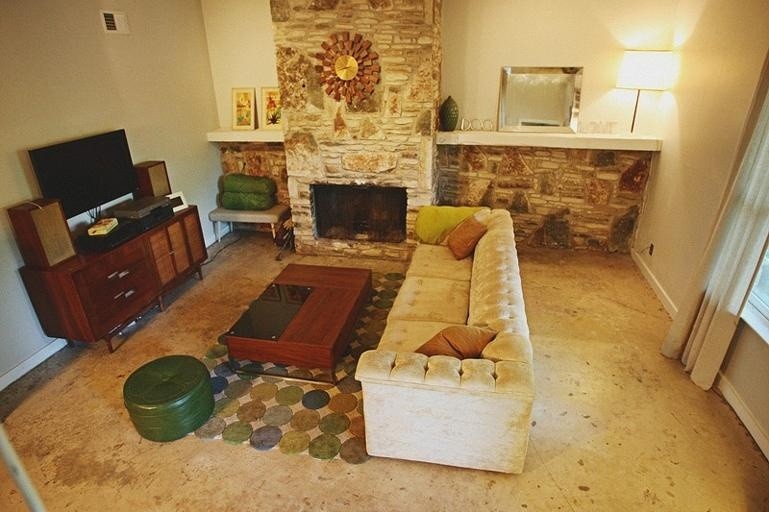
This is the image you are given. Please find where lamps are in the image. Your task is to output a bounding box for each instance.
[618,50,674,134]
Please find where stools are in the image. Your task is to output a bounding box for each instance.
[124,355,218,442]
[210,206,286,242]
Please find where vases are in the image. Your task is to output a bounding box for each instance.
[438,96,458,132]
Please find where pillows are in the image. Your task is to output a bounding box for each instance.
[443,218,484,260]
[415,326,498,361]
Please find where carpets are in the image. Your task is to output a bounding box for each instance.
[190,269,406,467]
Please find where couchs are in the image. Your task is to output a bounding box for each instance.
[357,206,542,476]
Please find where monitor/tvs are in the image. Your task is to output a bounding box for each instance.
[26,128,139,221]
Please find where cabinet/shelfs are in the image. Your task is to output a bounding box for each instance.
[136,207,208,292]
[71,239,163,342]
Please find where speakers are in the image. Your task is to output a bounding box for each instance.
[133,159,172,199]
[6,198,79,271]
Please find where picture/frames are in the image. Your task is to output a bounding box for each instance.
[496,65,584,134]
[232,87,254,131]
[166,190,189,212]
[260,86,281,132]
